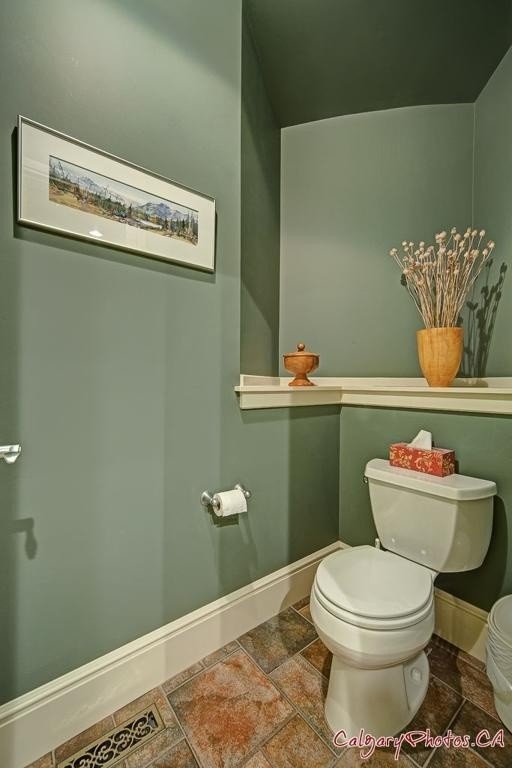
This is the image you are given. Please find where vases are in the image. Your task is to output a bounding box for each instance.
[414,328,465,388]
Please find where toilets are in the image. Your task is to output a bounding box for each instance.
[309,458,498,746]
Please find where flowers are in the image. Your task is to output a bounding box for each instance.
[389,225,497,328]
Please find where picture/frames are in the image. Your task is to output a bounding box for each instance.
[15,114,217,275]
